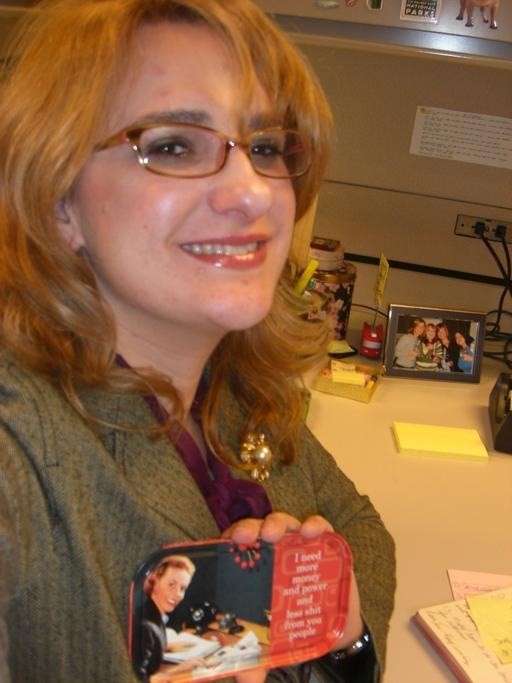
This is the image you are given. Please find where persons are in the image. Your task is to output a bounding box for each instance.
[131,555,196,683]
[0,0,398,683]
[394,318,474,373]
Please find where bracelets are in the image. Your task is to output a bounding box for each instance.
[331,632,370,661]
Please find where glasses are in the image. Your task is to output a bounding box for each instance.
[93,118,314,177]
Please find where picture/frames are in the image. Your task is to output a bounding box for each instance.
[384,305,487,385]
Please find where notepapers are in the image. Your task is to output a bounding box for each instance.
[392,420,488,461]
